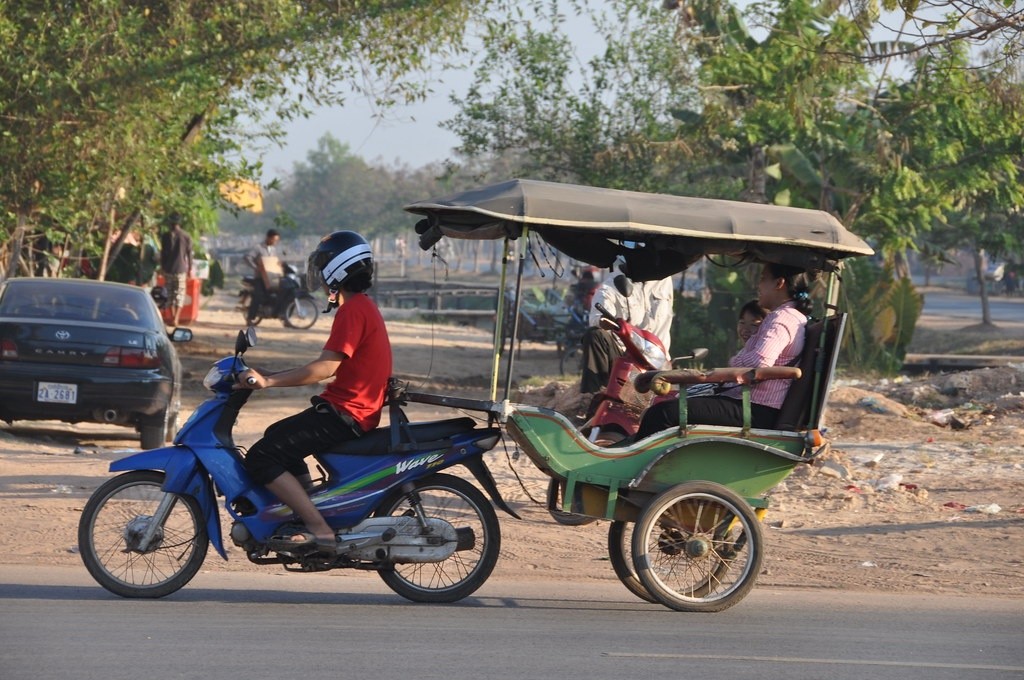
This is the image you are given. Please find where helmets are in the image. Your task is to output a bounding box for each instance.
[306,231,374,290]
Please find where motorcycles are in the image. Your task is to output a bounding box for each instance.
[234,251,320,331]
[73,325,523,603]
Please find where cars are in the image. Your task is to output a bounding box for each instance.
[0,274,196,451]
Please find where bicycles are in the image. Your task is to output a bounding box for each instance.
[559,310,590,378]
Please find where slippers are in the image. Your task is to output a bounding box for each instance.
[267,532,337,549]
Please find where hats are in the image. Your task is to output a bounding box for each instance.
[169,212,180,223]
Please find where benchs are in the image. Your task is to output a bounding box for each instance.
[773,322,836,432]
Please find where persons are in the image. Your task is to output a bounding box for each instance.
[634,262,814,445]
[101,215,160,294]
[245,229,285,326]
[229,231,392,549]
[581,240,675,396]
[160,212,192,326]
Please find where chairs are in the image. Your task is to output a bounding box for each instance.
[99,306,140,325]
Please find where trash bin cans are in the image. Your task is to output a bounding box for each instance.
[158,277,200,325]
